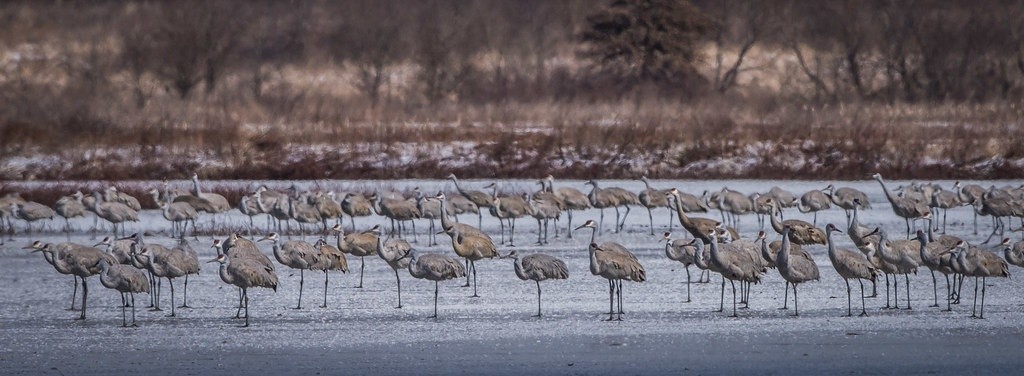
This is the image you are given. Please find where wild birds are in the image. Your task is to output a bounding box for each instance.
[0,172,1024,329]
